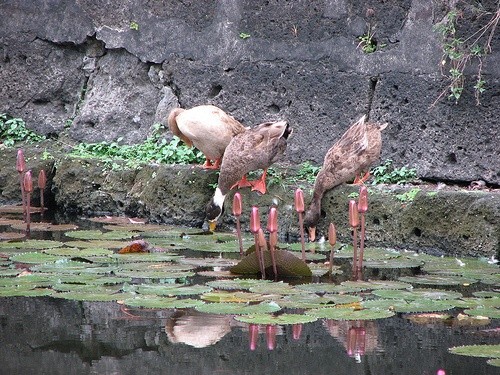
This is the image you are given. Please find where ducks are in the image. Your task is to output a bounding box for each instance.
[304,114,382,242]
[205,120,294,231]
[168,105,246,170]
